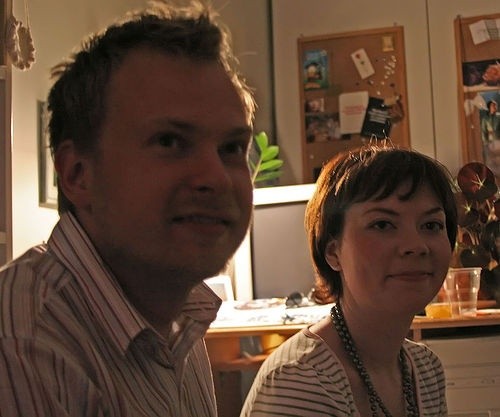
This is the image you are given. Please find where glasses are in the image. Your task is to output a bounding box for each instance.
[283,288,317,308]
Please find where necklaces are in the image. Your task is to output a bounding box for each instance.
[330,303,418,417]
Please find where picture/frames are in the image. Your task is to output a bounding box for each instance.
[36,100,59,210]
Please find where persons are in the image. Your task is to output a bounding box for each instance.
[240,129,456,417]
[0,0,258,417]
[481,100,500,146]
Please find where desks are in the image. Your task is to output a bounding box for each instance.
[204,301,500,416]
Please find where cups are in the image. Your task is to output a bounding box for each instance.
[425,279,452,320]
[447,267,483,318]
